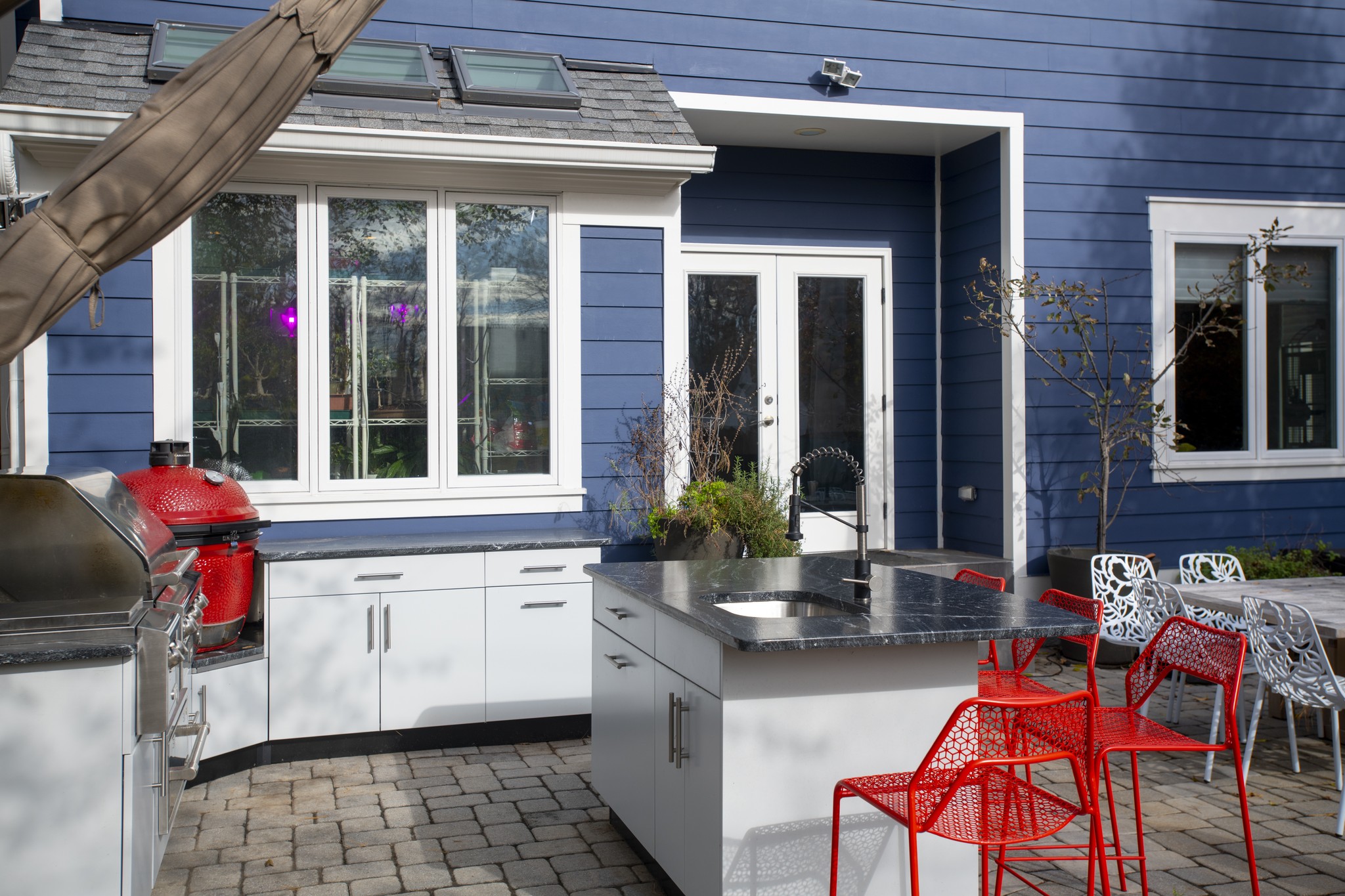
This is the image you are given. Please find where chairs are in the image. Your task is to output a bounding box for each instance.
[1126,575,1304,783]
[1231,595,1345,838]
[828,689,1114,896]
[948,566,1016,766]
[988,614,1264,896]
[974,586,1108,838]
[1085,550,1194,730]
[1170,553,1291,746]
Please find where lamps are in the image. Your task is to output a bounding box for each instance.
[956,484,979,503]
[817,56,864,97]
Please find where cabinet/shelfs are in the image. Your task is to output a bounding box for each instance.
[190,261,548,479]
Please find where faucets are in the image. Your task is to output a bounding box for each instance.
[781,446,884,602]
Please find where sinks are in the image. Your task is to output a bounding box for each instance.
[698,588,872,620]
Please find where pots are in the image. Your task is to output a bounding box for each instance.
[113,441,271,657]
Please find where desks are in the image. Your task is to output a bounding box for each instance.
[1156,576,1345,772]
